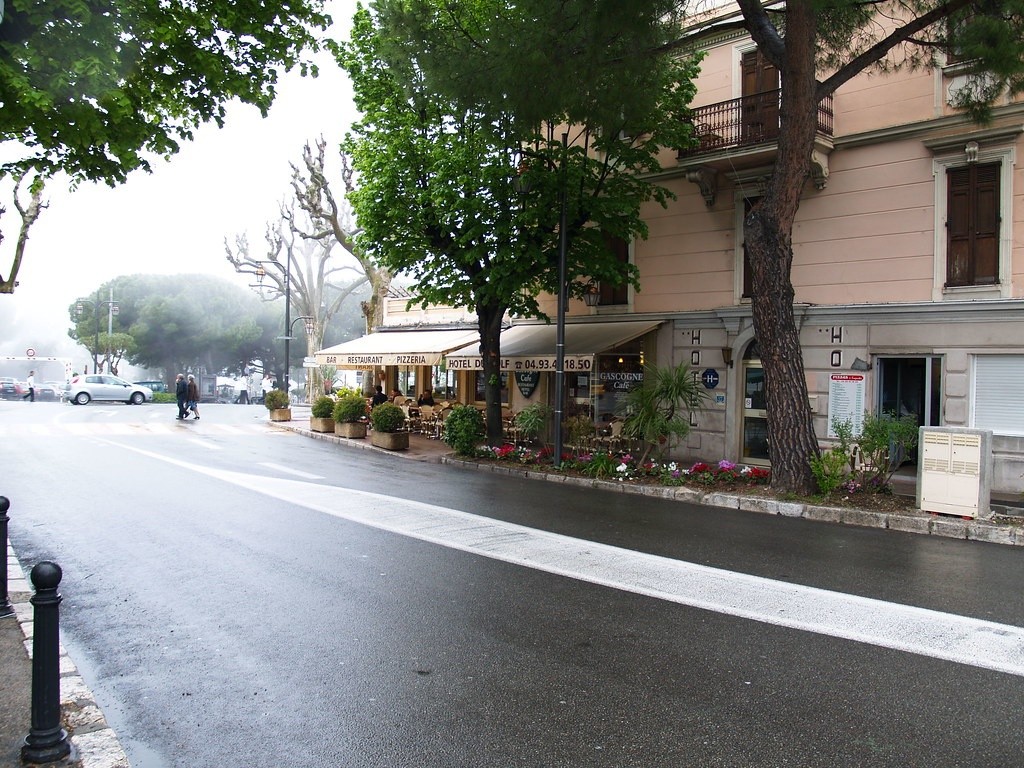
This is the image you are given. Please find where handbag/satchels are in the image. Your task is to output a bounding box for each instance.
[189,401,197,410]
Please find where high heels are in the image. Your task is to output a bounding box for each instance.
[193,416,200,420]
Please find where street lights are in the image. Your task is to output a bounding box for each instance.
[256,260,315,397]
[515,150,569,466]
[76,299,119,374]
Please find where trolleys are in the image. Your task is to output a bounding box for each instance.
[251,388,265,404]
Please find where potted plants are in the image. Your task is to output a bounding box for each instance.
[331,395,366,437]
[371,402,409,449]
[265,390,291,420]
[310,396,334,432]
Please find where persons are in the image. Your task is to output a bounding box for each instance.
[417,389,435,407]
[175,373,191,419]
[371,385,388,409]
[262,374,270,404]
[184,374,200,419]
[23,370,36,402]
[234,374,252,405]
[390,388,403,402]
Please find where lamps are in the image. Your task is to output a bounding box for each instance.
[722,347,733,368]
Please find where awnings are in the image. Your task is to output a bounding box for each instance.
[445,321,664,371]
[313,328,505,365]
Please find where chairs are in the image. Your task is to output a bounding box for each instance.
[400,399,530,448]
[578,414,637,452]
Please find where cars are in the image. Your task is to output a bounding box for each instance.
[0,377,66,402]
[62,375,153,405]
[133,381,165,394]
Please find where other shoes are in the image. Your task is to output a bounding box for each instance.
[176,417,183,419]
[185,412,190,418]
